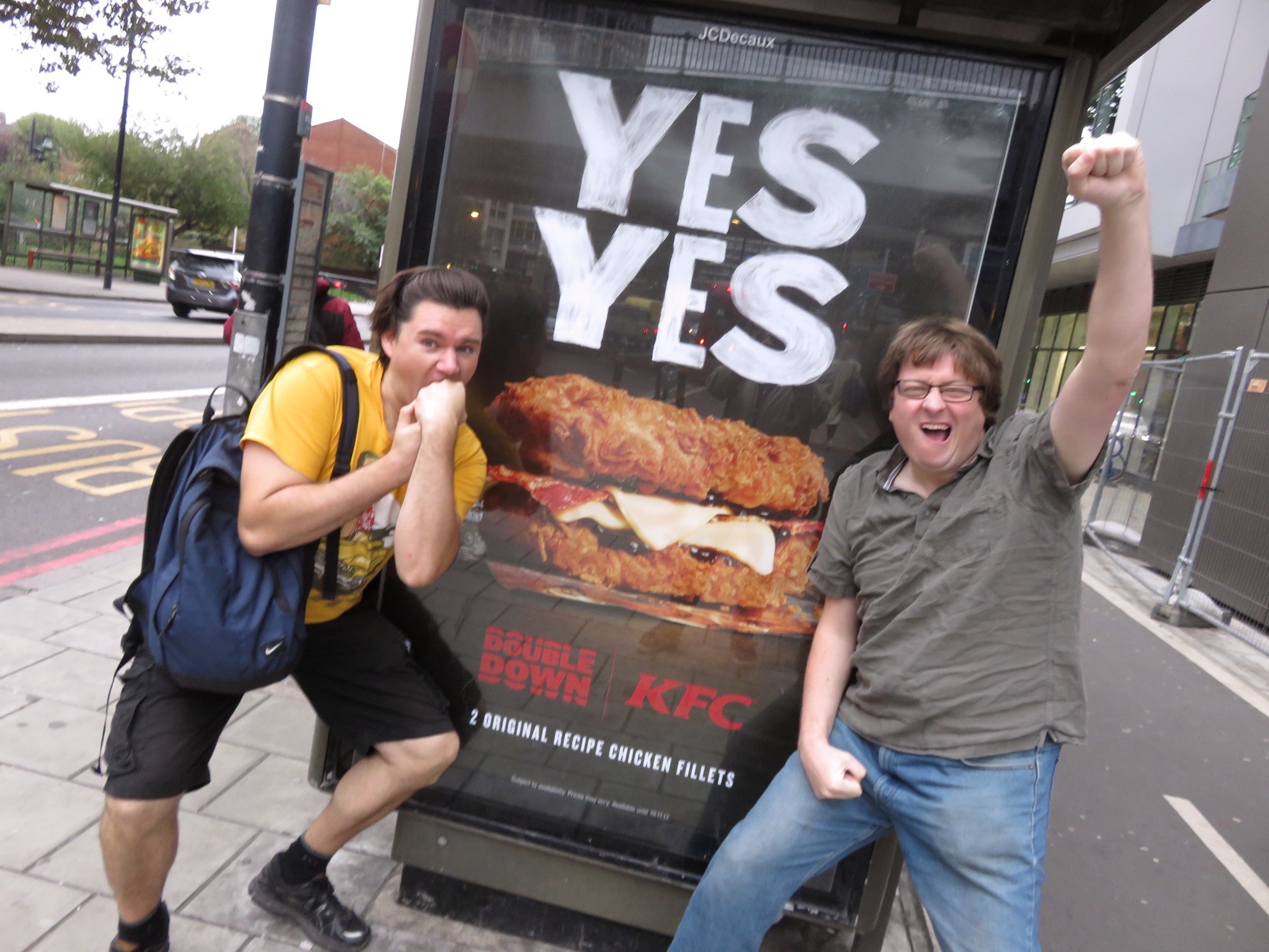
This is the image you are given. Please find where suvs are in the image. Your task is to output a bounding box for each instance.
[164,248,245,319]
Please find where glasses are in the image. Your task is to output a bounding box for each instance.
[893,379,986,403]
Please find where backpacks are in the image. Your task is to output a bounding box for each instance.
[115,340,360,695]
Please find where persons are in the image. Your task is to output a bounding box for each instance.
[97,266,487,952]
[666,130,1153,951]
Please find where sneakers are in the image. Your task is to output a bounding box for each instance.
[111,901,169,952]
[248,853,370,951]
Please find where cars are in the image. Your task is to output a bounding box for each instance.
[1095,410,1165,483]
